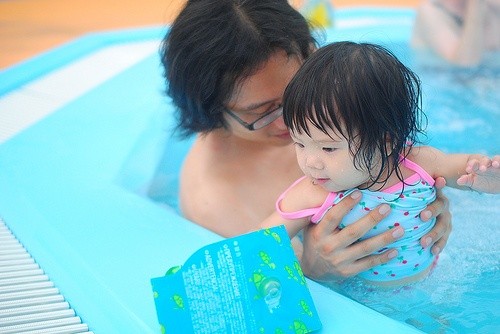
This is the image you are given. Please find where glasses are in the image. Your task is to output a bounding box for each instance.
[221,105,285,132]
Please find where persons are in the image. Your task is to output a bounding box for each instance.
[253,40,500,287]
[159,0,453,284]
[413,0,500,69]
